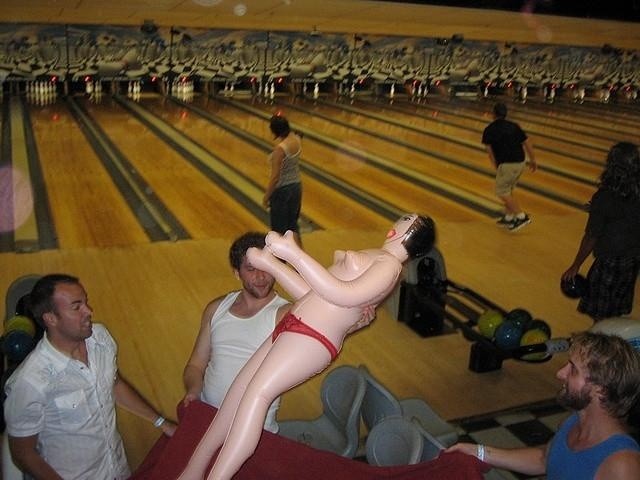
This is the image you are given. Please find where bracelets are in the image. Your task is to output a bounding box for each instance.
[477,442,485,461]
[153,415,165,427]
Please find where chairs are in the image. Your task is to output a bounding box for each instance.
[274,363,464,468]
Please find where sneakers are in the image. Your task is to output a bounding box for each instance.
[495,214,515,226]
[508,213,532,233]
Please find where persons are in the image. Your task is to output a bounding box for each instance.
[560,140,639,322]
[480,102,537,232]
[178,211,435,480]
[4,272,179,479]
[262,114,304,263]
[181,229,377,435]
[445,328,639,479]
